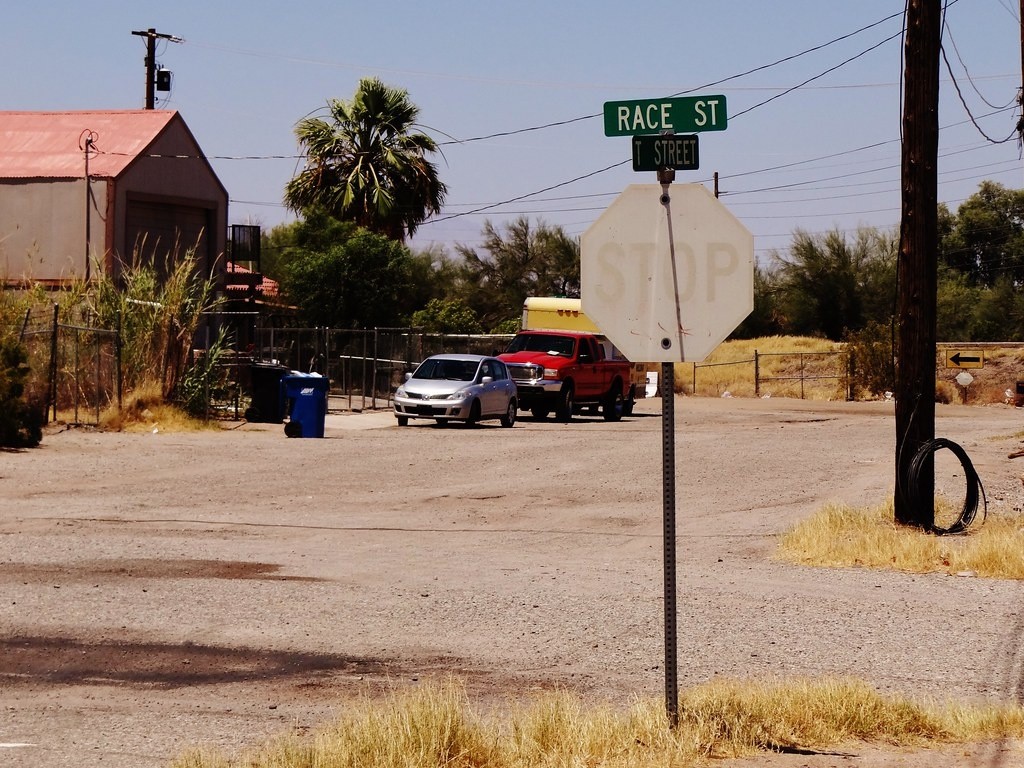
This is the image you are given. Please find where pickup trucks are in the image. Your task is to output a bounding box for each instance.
[479,329,629,424]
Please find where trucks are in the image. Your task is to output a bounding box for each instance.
[523,297,648,418]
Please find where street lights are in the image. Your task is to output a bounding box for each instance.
[132,28,185,110]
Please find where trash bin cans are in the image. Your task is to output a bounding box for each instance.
[283,374,332,438]
[244,363,287,425]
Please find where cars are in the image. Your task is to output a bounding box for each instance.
[393,354,519,429]
